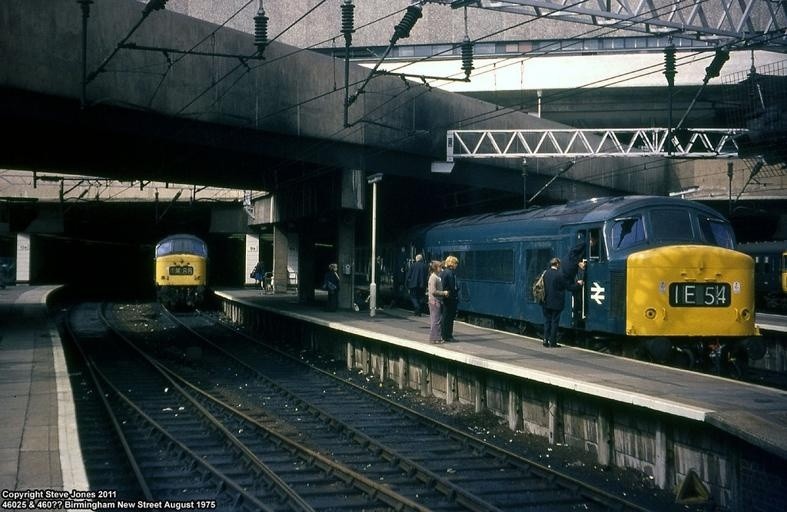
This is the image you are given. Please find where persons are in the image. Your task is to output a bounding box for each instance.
[536,256,583,348]
[326,263,341,312]
[254,257,265,290]
[438,255,462,342]
[404,254,429,317]
[427,260,450,344]
[368,255,385,311]
[561,236,598,317]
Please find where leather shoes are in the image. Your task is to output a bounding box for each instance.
[414,308,422,317]
[430,336,459,344]
[543,339,561,347]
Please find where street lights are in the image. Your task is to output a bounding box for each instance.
[367,173,383,317]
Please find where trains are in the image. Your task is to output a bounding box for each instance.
[355,194,767,382]
[737,240,787,317]
[153,233,209,309]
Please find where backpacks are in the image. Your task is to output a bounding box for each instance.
[533,268,548,305]
